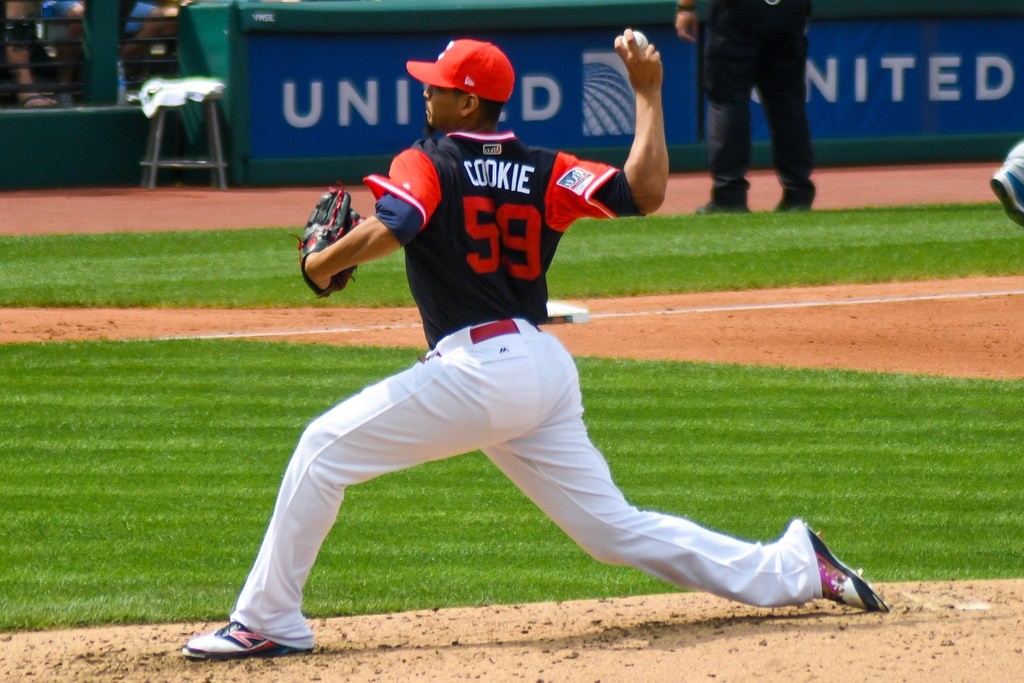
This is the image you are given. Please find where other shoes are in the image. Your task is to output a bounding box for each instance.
[695,201,747,216]
[773,181,815,212]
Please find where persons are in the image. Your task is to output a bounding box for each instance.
[178,29,891,662]
[989,140,1024,226]
[0,0,84,108]
[674,0,816,215]
[119,0,192,90]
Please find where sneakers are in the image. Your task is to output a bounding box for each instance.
[798,518,890,612]
[181,621,313,661]
[990,167,1024,228]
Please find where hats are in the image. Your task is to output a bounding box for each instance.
[406,40,515,103]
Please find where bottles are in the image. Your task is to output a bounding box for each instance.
[116,62,126,104]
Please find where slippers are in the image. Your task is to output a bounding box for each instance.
[19,93,59,108]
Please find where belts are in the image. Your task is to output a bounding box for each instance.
[417,319,542,364]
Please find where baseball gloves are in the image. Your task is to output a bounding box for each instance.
[298,184,370,299]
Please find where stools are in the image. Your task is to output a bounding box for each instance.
[139,87,229,192]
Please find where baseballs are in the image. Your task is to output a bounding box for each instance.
[624,30,649,52]
